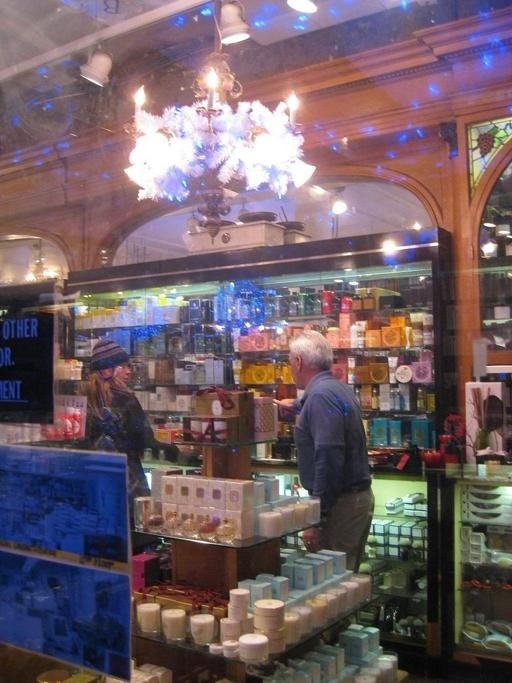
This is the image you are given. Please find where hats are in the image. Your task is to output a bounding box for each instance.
[88,340,129,371]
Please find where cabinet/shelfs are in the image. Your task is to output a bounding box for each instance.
[0,258,512,683]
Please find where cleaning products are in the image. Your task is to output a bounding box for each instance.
[298,284,307,315]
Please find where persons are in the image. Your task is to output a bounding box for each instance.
[3,461,125,669]
[277,328,376,643]
[75,338,191,524]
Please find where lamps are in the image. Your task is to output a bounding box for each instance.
[285,0,318,17]
[124,66,317,246]
[214,3,256,47]
[25,239,60,281]
[81,34,117,92]
[331,186,347,216]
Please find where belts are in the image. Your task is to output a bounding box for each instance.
[307,479,371,497]
